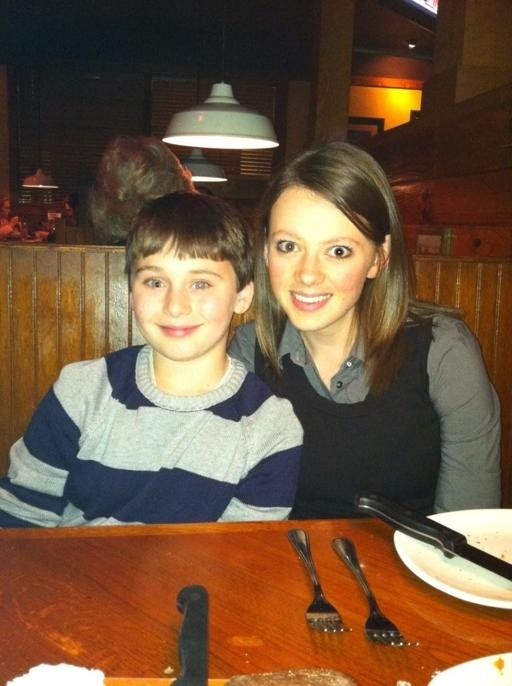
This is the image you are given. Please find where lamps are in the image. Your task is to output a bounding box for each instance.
[161,1,281,151]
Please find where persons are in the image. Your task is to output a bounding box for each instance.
[86,132,205,245]
[34,191,89,244]
[221,139,504,523]
[0,196,29,241]
[1,189,307,527]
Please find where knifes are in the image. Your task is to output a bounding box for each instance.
[349,489,512,585]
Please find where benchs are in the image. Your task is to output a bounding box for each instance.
[0,241,511,507]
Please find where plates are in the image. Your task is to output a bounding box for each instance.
[392,508,512,610]
[426,651,512,686]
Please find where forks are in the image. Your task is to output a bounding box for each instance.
[282,526,353,636]
[331,536,421,650]
